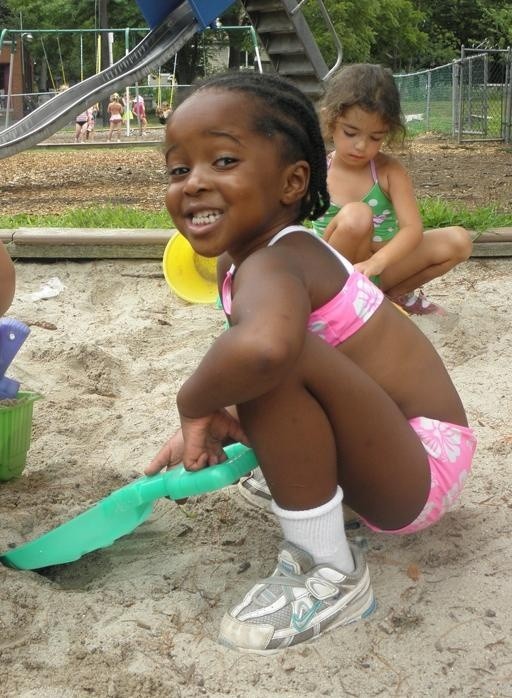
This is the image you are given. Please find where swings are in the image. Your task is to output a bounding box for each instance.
[158,52,178,125]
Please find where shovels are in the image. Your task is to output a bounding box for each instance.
[1,441,258,570]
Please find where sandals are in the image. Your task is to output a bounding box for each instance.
[395,288,447,319]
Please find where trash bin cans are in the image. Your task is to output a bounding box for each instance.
[144,95,153,115]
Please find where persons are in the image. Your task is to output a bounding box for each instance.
[70,89,173,144]
[304,61,473,322]
[144,70,479,654]
[1,241,18,320]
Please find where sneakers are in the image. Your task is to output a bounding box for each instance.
[219,536,376,657]
[236,475,274,515]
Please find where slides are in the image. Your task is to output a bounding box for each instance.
[0,0,201,160]
